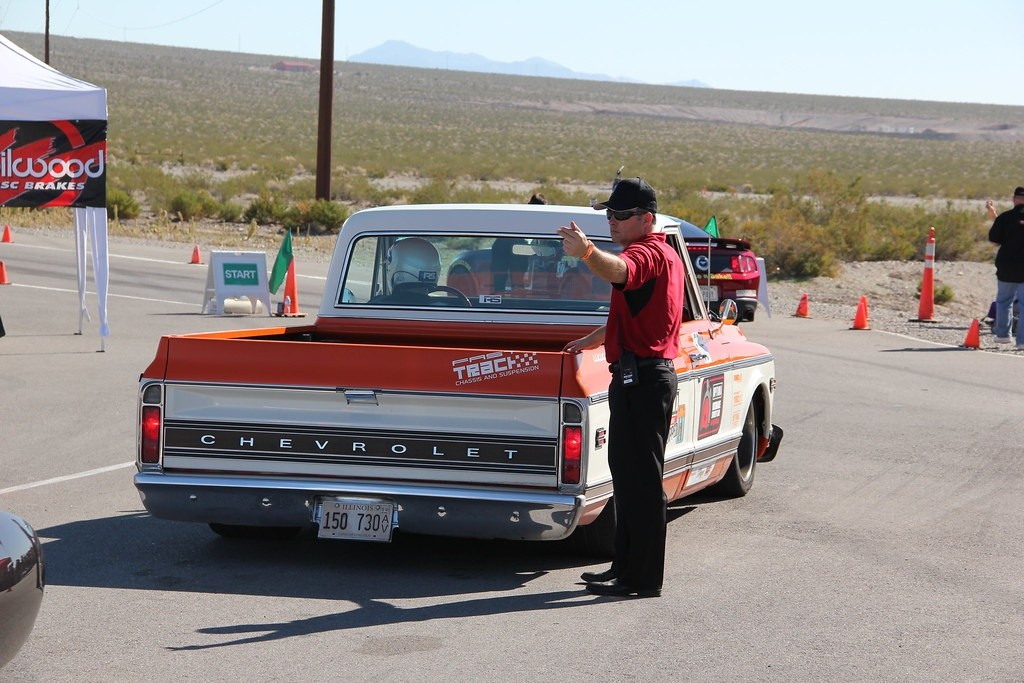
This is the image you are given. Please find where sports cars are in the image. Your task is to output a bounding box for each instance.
[446,218,761,322]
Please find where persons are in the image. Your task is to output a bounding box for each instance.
[988,187,1024,350]
[557,178,686,599]
[365,239,442,308]
[528,193,548,204]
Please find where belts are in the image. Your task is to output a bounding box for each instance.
[608,358,665,373]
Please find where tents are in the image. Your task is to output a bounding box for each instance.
[0,35,109,352]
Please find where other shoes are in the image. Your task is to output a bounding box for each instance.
[1017,343,1024,349]
[994,336,1011,343]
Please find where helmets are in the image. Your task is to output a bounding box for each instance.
[386,238,441,296]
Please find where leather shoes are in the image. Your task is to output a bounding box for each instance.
[580,567,618,583]
[586,576,662,598]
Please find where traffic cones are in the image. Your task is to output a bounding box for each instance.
[272,254,308,317]
[959,319,981,350]
[791,292,813,319]
[0,260,11,285]
[188,246,204,264]
[0,225,14,243]
[849,296,872,329]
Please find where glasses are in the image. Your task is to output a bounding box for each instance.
[607,210,642,221]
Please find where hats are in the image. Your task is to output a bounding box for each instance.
[1015,187,1024,196]
[592,176,657,213]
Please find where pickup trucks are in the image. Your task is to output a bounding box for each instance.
[131,203,783,540]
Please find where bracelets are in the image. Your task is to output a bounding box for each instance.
[580,239,594,260]
